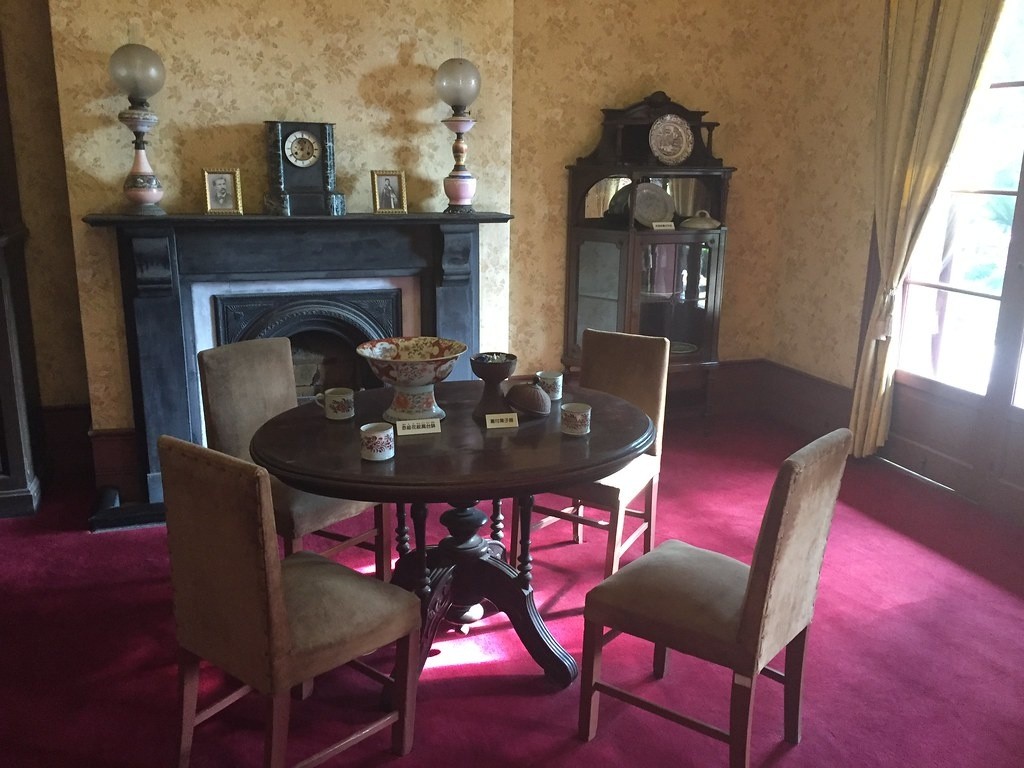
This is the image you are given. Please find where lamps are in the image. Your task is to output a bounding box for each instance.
[107,42,168,216]
[431,58,483,216]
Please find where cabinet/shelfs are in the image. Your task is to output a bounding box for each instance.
[560,91,737,419]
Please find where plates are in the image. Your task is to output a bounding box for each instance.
[649,113,695,165]
[639,292,680,299]
[628,182,675,227]
[668,341,698,354]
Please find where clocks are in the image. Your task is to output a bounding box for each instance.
[262,120,347,219]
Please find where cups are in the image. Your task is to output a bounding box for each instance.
[536,370,563,401]
[560,403,592,436]
[359,422,395,461]
[315,387,355,419]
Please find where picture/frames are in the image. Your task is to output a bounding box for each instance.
[370,169,407,215]
[198,167,244,216]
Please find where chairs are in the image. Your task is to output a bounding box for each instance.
[157,434,424,768]
[577,425,854,767]
[509,328,672,578]
[197,337,391,581]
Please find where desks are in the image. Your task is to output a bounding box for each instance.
[249,379,656,717]
